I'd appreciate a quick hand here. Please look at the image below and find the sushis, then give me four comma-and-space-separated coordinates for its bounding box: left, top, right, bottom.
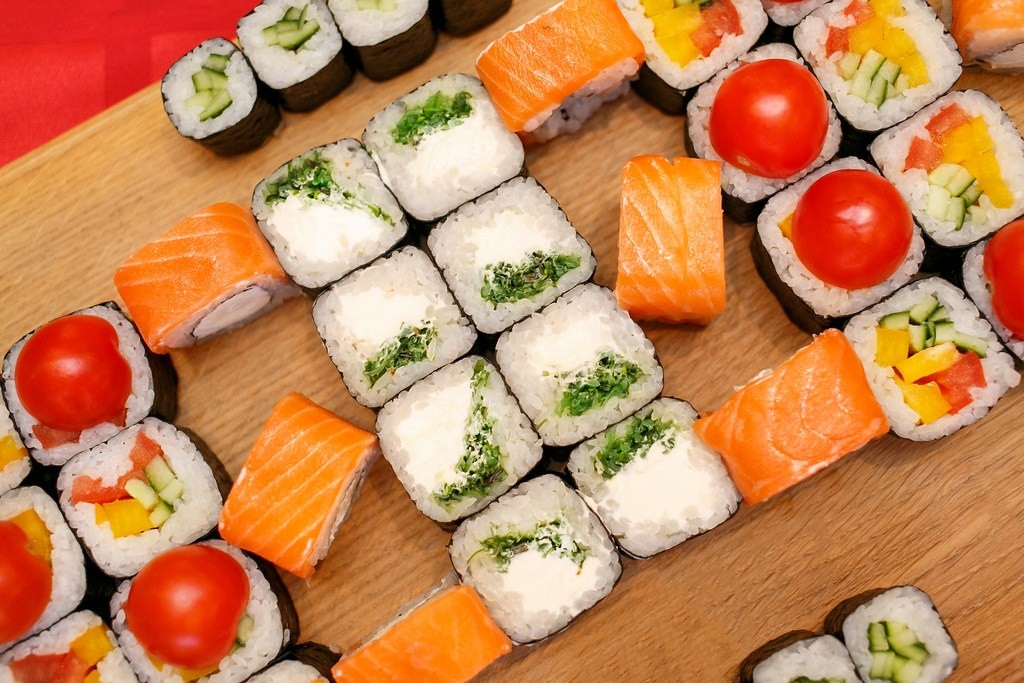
0, 0, 1024, 683
